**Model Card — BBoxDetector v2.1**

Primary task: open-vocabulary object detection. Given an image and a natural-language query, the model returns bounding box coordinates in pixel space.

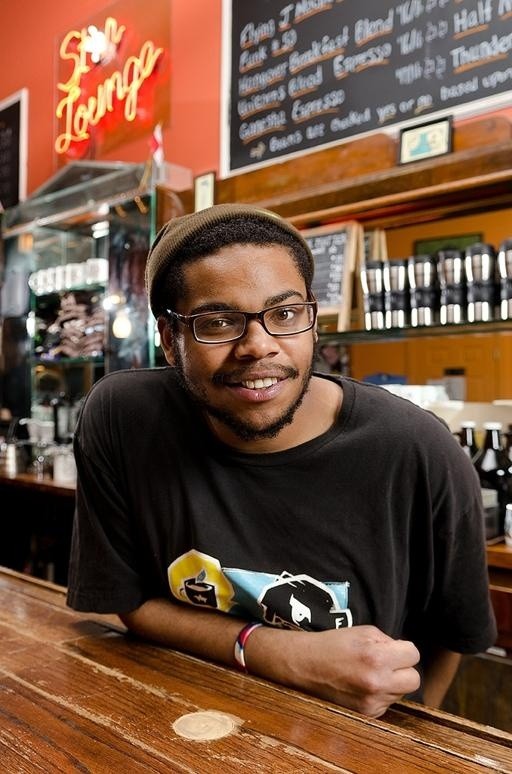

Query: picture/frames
[191,171,219,215]
[400,112,453,164]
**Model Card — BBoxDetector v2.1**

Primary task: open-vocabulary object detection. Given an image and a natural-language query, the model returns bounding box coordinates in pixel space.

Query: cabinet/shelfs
[278,173,510,565]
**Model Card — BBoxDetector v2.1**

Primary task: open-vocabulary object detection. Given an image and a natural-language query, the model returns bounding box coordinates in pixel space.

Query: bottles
[456,420,512,548]
[359,242,512,332]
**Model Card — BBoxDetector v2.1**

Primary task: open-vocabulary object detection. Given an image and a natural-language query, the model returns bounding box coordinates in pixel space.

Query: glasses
[162,294,320,344]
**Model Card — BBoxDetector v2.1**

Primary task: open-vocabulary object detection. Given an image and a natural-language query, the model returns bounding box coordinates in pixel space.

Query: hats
[146,203,316,317]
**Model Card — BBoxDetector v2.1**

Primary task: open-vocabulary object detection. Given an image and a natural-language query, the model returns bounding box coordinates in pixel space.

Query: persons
[68,205,500,721]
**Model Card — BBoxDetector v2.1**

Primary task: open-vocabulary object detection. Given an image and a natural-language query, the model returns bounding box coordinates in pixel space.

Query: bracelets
[234,620,264,676]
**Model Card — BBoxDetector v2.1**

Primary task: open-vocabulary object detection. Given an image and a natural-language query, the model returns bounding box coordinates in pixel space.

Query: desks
[0,568,512,773]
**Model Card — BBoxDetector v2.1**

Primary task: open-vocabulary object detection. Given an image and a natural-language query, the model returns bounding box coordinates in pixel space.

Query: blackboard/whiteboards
[218,0,512,182]
[298,219,379,317]
